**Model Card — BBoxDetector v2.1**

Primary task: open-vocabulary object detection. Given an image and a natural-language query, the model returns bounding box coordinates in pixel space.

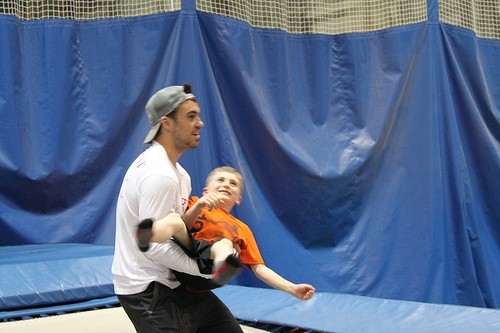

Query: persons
[136,166,315,301]
[111,83,245,333]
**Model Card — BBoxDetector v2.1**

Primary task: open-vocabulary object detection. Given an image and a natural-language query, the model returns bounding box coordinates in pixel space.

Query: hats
[144,86,196,144]
